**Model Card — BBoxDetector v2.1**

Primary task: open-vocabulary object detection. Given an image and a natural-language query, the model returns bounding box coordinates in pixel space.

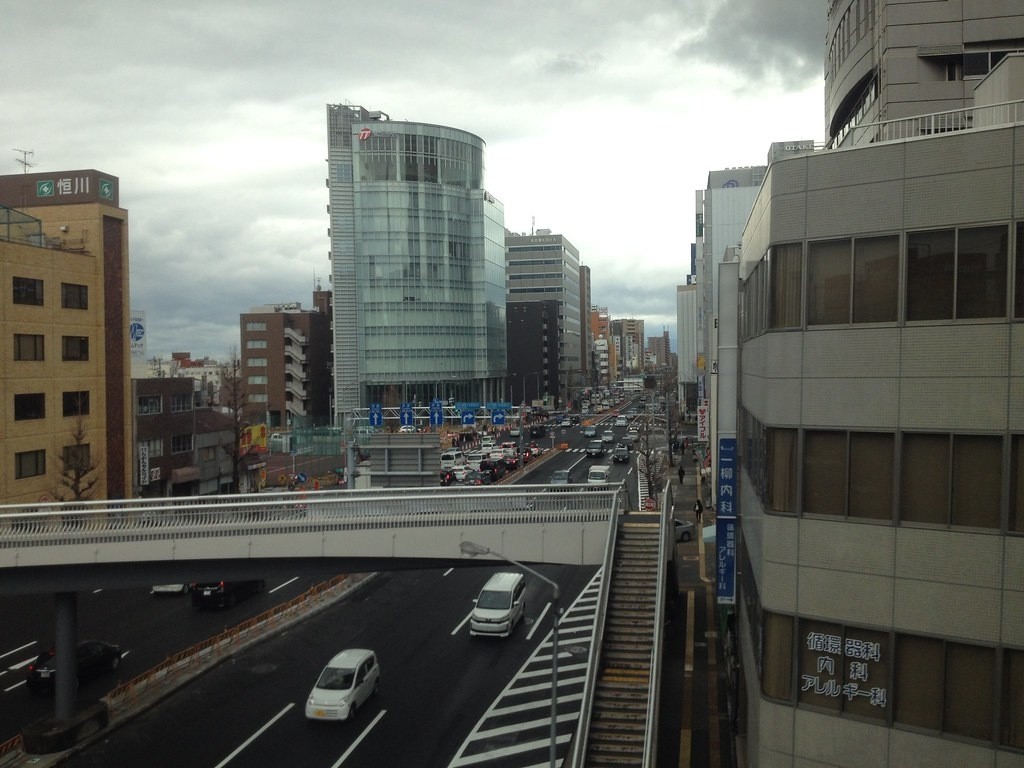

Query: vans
[305,648,380,723]
[469,573,528,638]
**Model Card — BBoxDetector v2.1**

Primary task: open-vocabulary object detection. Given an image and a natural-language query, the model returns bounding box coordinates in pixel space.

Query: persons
[678,466,685,484]
[674,437,698,461]
[693,499,704,523]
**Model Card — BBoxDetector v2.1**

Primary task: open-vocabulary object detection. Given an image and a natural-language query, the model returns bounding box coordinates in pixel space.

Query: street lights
[436,376,457,401]
[522,371,537,404]
[457,537,558,768]
[501,373,517,403]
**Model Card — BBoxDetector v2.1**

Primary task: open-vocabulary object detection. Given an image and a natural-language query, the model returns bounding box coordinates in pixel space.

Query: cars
[674,519,695,542]
[585,425,596,437]
[600,430,616,442]
[549,470,573,484]
[558,390,654,464]
[190,575,266,605]
[438,425,549,485]
[586,440,606,459]
[27,641,118,696]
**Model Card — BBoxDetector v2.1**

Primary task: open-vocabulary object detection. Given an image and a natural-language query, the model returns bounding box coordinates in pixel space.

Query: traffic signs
[401,403,412,426]
[430,402,444,427]
[369,404,384,428]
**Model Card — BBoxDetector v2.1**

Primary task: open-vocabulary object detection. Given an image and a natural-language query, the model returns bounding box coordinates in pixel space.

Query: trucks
[588,465,611,483]
